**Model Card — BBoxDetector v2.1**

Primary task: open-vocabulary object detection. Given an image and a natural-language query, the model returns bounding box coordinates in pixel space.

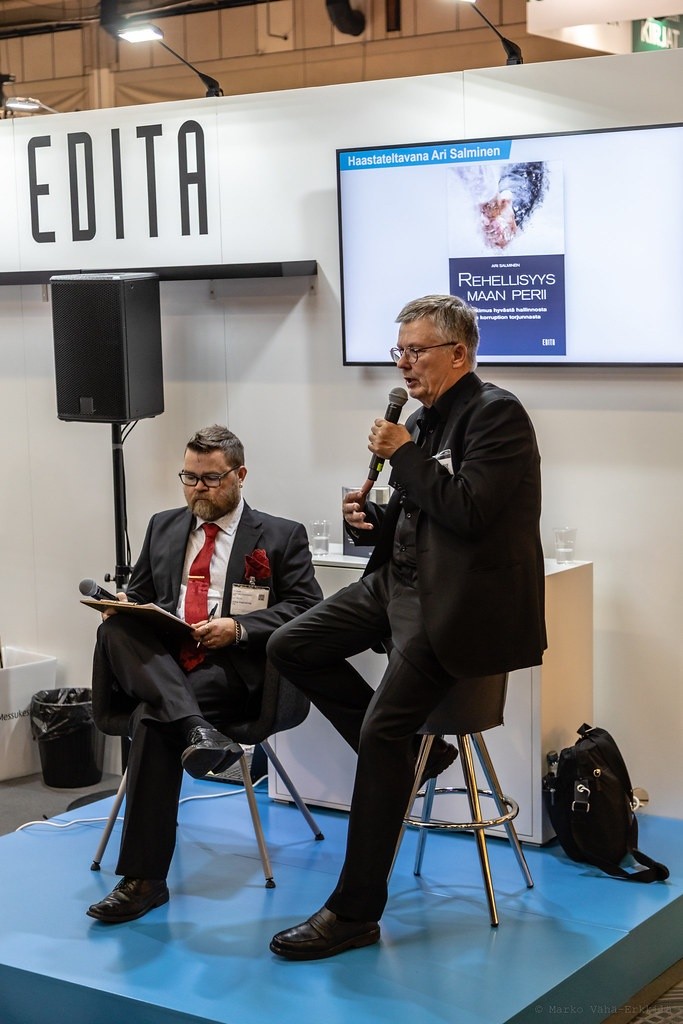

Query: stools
[386,672,534,927]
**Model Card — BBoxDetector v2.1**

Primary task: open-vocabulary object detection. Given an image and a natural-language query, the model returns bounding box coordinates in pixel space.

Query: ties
[180,522,223,672]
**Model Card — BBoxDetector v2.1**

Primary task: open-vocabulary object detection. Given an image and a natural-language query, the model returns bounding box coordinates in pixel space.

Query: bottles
[543,772,558,807]
[546,751,560,778]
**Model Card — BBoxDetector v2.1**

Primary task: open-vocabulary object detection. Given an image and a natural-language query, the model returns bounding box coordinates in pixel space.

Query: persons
[84,425,323,924]
[266,293,549,964]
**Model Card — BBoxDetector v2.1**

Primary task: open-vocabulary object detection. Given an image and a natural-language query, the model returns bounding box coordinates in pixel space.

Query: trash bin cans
[31,688,106,793]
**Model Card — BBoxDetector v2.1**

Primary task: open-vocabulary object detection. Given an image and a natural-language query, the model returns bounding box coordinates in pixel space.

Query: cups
[555,526,577,564]
[311,521,328,555]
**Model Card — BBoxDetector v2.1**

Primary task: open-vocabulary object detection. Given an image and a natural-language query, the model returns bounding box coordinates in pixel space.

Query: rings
[208,640,212,646]
[206,625,210,633]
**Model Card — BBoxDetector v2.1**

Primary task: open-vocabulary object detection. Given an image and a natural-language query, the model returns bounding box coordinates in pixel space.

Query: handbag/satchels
[541,722,670,883]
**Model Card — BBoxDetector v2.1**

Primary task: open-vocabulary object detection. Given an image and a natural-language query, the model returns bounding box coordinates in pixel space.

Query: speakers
[49,272,164,421]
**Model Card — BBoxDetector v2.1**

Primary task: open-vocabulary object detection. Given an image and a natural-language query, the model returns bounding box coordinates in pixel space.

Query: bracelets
[234,621,240,646]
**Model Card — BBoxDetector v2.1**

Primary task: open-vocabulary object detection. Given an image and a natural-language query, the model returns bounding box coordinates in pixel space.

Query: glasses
[389,343,457,363]
[178,464,242,487]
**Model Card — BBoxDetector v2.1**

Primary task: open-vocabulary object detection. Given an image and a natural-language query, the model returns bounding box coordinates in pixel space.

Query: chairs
[89,642,325,889]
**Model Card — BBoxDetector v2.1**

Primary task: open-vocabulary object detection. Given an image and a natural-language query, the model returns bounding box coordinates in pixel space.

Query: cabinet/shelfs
[266,542,594,847]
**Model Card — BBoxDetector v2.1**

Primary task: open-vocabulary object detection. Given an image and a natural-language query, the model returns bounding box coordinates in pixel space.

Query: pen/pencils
[196,603,219,649]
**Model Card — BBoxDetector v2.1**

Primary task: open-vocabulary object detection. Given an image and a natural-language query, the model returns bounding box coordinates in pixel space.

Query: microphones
[79,580,119,601]
[367,387,408,481]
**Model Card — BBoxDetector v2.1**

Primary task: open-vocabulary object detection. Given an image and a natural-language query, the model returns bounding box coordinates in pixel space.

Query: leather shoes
[86,875,169,921]
[415,734,458,792]
[269,906,381,960]
[180,726,244,779]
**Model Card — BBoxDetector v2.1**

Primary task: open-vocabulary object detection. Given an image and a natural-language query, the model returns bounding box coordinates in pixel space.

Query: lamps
[117,23,223,97]
[6,97,60,114]
